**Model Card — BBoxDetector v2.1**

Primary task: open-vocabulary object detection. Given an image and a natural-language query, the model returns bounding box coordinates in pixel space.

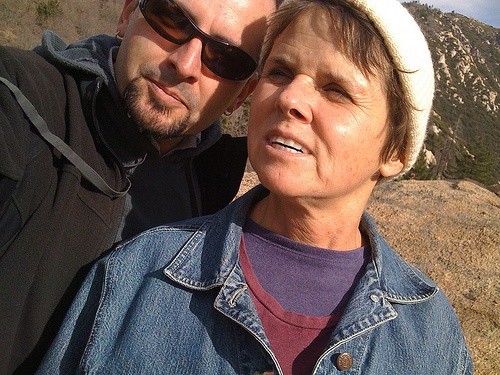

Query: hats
[259,0,436,177]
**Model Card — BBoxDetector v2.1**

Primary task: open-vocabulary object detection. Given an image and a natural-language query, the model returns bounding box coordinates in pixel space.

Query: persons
[0,0,282,375]
[35,0,473,375]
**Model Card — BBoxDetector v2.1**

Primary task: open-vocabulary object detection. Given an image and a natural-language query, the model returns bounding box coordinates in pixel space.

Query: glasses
[136,0,261,81]
